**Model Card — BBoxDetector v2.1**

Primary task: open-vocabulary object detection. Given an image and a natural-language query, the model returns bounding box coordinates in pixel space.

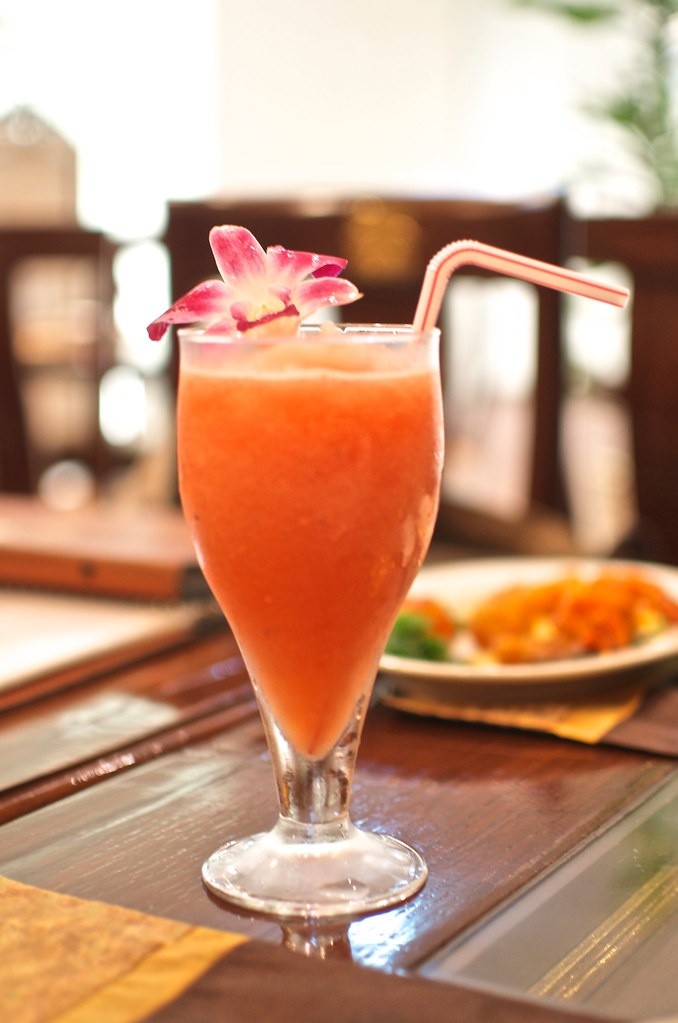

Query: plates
[352,557,678,696]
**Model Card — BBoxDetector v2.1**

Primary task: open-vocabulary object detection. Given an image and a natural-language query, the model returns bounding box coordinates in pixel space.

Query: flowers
[144,223,365,341]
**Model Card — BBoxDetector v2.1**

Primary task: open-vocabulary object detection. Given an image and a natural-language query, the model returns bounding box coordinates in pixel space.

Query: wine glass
[173,323,445,922]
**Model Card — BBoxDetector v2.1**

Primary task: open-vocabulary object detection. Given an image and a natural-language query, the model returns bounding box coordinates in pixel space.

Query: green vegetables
[386,614,453,661]
